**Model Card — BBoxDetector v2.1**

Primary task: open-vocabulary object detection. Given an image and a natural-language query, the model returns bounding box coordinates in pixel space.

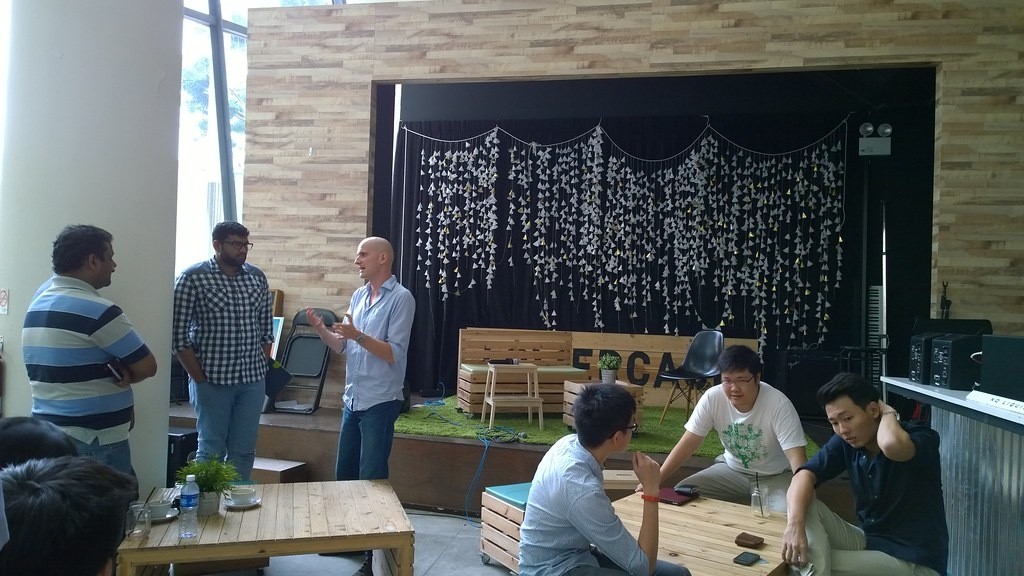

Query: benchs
[457,329,590,419]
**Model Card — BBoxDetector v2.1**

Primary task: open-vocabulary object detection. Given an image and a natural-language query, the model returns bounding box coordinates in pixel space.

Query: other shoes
[351,561,373,576]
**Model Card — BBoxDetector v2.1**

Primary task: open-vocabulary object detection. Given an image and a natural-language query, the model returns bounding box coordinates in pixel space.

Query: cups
[231,488,256,504]
[173,495,180,507]
[147,501,171,517]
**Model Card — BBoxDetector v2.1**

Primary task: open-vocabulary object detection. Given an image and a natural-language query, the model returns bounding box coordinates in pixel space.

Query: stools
[479,482,532,576]
[480,362,544,431]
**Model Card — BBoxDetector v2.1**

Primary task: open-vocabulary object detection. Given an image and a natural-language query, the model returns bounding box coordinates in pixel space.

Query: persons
[781,372,949,576]
[0,417,139,576]
[172,221,275,482]
[305,237,416,576]
[22,225,157,501]
[635,345,808,514]
[517,383,692,576]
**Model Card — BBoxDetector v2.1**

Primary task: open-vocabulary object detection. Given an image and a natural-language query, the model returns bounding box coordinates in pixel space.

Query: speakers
[167,426,198,487]
[785,349,863,421]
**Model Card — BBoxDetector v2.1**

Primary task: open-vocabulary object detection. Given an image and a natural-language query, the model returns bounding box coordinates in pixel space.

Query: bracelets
[641,495,659,502]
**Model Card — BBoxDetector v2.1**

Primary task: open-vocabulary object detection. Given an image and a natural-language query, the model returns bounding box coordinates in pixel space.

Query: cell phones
[733,552,760,566]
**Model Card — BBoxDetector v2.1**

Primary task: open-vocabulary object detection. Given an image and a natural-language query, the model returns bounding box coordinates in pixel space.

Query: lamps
[858,123,892,155]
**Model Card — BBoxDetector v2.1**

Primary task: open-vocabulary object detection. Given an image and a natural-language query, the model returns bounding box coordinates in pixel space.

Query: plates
[223,497,262,509]
[145,508,180,522]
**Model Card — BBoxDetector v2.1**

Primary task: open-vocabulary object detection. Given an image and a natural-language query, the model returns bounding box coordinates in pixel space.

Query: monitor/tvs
[981,334,1024,402]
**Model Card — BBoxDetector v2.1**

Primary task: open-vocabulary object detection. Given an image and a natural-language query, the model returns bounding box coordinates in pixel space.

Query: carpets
[394,394,820,463]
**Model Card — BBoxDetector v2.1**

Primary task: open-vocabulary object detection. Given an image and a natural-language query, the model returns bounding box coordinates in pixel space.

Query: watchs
[881,410,901,423]
[356,333,365,346]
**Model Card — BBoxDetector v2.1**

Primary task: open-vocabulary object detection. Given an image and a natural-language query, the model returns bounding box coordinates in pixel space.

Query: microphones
[487,359,519,364]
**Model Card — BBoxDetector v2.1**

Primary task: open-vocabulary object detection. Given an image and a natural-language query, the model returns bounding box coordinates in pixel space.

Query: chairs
[269,308,338,415]
[658,331,724,426]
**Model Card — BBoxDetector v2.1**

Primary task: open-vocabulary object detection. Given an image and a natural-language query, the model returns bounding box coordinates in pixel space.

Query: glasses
[722,373,756,386]
[218,239,254,249]
[609,421,639,439]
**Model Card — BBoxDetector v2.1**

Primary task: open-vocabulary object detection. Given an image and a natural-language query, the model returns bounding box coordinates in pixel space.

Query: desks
[563,380,644,439]
[611,490,800,576]
[115,479,415,576]
[879,375,1024,576]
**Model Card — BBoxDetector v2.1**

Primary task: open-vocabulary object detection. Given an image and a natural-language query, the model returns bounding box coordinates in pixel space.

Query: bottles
[126,500,151,539]
[178,474,199,537]
[751,486,771,518]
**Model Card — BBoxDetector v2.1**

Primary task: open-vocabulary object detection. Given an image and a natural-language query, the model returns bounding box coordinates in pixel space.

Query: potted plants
[173,450,243,516]
[597,352,621,384]
